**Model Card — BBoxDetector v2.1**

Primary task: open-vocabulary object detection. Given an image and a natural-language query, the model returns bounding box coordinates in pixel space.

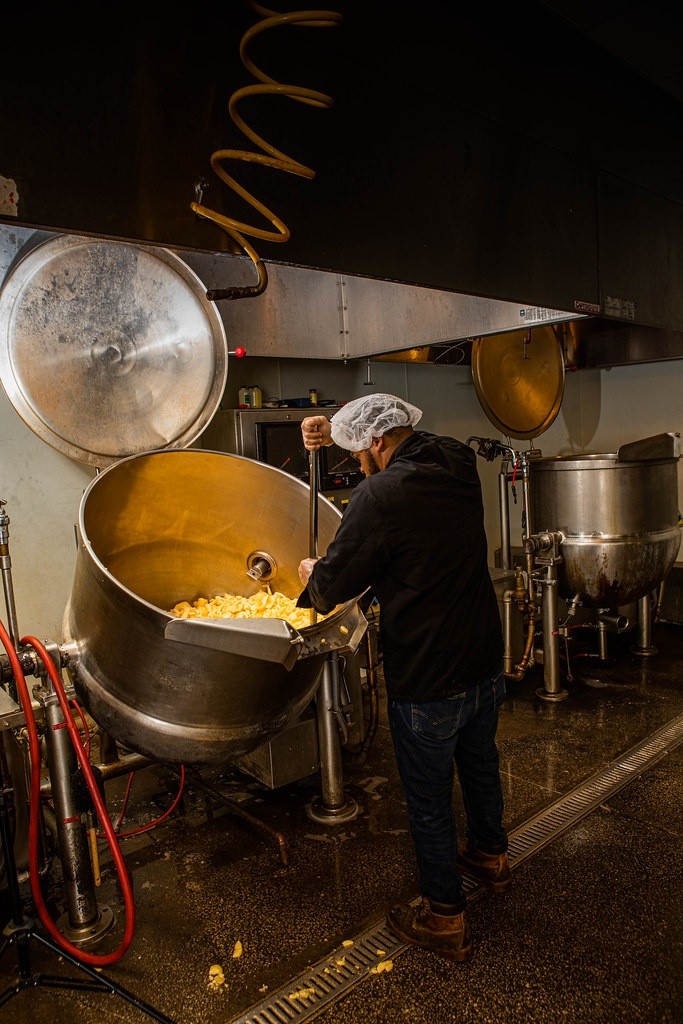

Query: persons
[295,393,514,963]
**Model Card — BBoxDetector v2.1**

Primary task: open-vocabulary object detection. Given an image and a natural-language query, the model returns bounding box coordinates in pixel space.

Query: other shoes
[456,839,514,892]
[387,896,473,962]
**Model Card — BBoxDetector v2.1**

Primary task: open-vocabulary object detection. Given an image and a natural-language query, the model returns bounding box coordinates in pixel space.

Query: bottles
[239,385,262,409]
